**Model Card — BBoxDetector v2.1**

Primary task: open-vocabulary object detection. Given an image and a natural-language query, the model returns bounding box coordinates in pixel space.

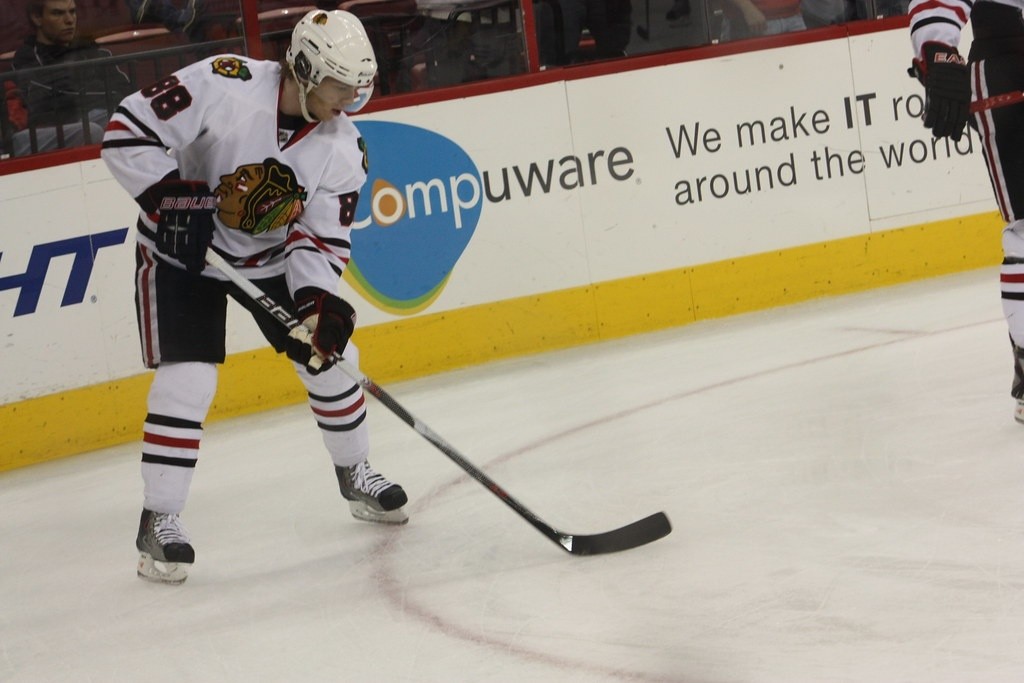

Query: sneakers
[1012,346,1024,425]
[334,458,409,525]
[136,508,195,585]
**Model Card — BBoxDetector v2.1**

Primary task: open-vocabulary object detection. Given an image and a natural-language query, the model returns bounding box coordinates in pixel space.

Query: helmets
[286,9,378,96]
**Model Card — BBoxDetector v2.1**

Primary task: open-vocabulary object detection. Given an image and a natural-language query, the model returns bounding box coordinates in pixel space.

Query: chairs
[0,0,427,131]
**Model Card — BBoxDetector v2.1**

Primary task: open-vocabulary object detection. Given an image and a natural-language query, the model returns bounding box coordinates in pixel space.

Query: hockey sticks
[970,90,1024,116]
[201,246,676,558]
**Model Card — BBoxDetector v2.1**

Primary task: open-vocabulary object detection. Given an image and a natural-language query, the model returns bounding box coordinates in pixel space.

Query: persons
[98,10,408,585]
[0,0,431,198]
[721,0,805,45]
[908,0,1024,429]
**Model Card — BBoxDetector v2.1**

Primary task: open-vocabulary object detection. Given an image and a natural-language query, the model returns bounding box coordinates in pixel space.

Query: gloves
[148,179,216,276]
[907,41,972,142]
[287,292,357,376]
